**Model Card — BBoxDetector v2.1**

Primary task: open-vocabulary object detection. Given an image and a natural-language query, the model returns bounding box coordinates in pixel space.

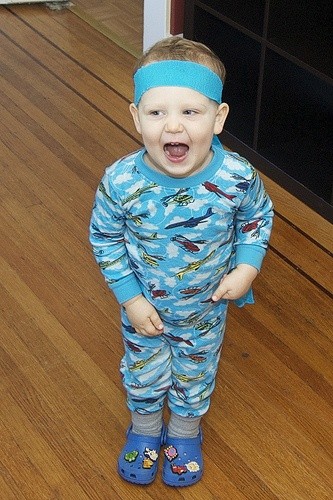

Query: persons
[88,36,274,484]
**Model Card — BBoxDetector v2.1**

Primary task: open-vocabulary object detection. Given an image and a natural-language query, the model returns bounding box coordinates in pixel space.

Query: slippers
[117,418,167,483]
[162,423,205,486]
